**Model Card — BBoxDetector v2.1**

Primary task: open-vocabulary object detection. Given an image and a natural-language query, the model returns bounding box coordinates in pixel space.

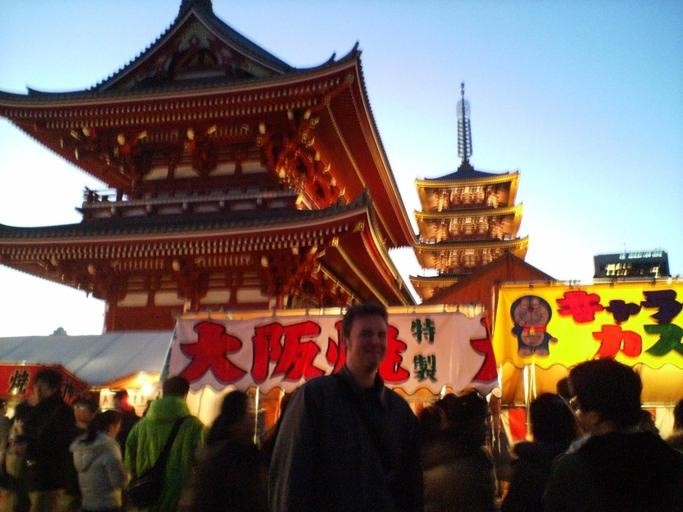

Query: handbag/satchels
[127,466,163,506]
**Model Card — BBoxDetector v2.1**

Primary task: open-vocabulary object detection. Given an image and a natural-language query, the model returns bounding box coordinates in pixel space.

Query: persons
[0,302,683,511]
